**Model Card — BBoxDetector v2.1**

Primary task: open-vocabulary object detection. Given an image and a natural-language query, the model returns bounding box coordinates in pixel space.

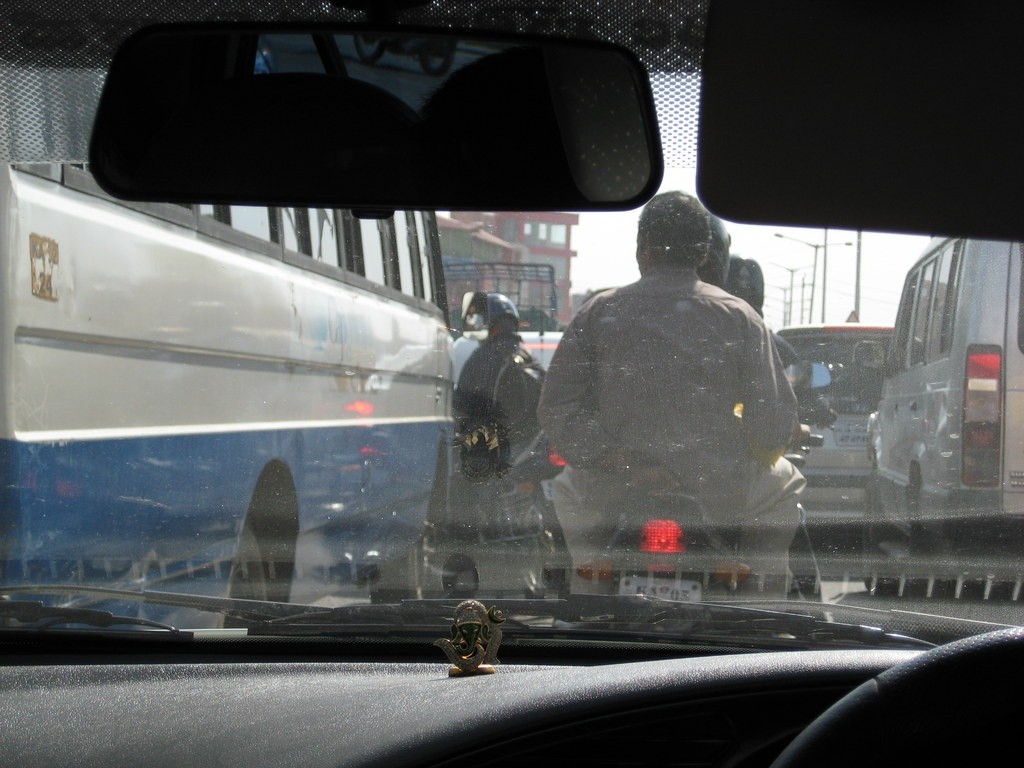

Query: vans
[866,228,1024,517]
[774,323,905,516]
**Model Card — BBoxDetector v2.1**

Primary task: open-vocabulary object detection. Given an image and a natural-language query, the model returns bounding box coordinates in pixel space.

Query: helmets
[636,190,712,270]
[487,292,520,332]
[706,214,730,287]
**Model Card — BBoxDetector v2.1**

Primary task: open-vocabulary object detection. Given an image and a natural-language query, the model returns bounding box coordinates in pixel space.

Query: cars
[449,324,573,386]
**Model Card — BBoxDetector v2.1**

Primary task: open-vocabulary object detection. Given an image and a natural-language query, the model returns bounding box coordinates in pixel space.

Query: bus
[2,42,473,636]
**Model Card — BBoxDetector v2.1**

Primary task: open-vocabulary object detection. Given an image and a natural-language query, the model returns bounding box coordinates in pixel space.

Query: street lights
[773,231,853,325]
[770,261,810,326]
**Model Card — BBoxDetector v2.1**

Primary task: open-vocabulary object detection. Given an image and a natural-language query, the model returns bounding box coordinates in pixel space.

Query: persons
[466,306,483,325]
[449,293,534,586]
[536,192,821,625]
